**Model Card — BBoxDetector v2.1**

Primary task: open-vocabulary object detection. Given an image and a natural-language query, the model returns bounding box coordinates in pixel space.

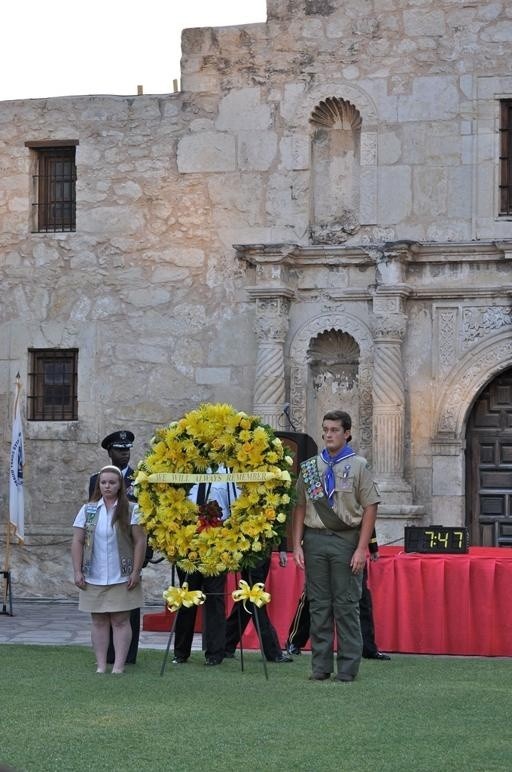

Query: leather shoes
[310,670,355,681]
[170,653,220,670]
[270,639,302,663]
[366,652,391,660]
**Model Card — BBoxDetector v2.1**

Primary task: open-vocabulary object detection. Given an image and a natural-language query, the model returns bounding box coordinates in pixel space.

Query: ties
[196,483,206,506]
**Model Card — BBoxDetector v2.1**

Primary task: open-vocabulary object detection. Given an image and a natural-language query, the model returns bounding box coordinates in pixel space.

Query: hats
[101,431,135,449]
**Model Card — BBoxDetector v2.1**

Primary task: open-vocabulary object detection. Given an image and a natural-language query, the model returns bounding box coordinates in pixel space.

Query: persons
[69,464,147,677]
[87,426,155,666]
[282,526,390,660]
[168,458,241,668]
[219,547,290,663]
[291,408,380,684]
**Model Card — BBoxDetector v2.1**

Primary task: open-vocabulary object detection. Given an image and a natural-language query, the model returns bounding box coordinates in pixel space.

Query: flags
[9,382,26,545]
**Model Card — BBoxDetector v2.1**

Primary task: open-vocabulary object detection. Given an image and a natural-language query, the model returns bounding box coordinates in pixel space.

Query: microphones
[281,402,291,415]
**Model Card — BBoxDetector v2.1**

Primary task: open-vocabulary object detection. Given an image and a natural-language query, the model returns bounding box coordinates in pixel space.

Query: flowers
[134,403,293,577]
